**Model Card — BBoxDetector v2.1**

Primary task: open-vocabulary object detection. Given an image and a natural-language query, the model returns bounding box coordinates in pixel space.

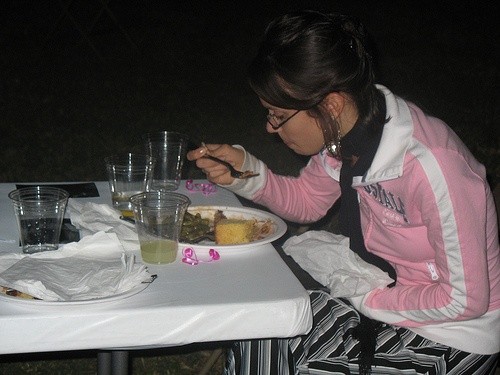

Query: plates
[143,205,288,249]
[0,253,155,304]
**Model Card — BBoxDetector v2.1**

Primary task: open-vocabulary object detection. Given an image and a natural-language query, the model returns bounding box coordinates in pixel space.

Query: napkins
[0,197,150,303]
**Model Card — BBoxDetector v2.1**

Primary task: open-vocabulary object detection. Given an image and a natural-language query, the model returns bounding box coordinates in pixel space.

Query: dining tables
[0,178,313,375]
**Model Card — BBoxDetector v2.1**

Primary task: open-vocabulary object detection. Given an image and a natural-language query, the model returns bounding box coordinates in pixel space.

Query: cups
[129,191,190,264]
[142,130,189,191]
[104,153,153,213]
[8,186,69,254]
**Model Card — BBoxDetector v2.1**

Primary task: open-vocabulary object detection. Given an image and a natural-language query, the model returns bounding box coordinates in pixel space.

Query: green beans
[146,210,217,244]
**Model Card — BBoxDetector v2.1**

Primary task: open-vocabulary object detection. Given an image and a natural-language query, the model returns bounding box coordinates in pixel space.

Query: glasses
[259,105,301,130]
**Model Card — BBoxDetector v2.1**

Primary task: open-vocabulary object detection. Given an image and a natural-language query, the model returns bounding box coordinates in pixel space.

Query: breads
[214,218,255,244]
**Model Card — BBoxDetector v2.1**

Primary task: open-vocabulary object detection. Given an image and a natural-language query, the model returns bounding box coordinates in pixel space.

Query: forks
[202,154,260,179]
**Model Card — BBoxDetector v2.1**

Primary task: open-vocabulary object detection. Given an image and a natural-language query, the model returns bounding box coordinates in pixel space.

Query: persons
[187,11,500,375]
